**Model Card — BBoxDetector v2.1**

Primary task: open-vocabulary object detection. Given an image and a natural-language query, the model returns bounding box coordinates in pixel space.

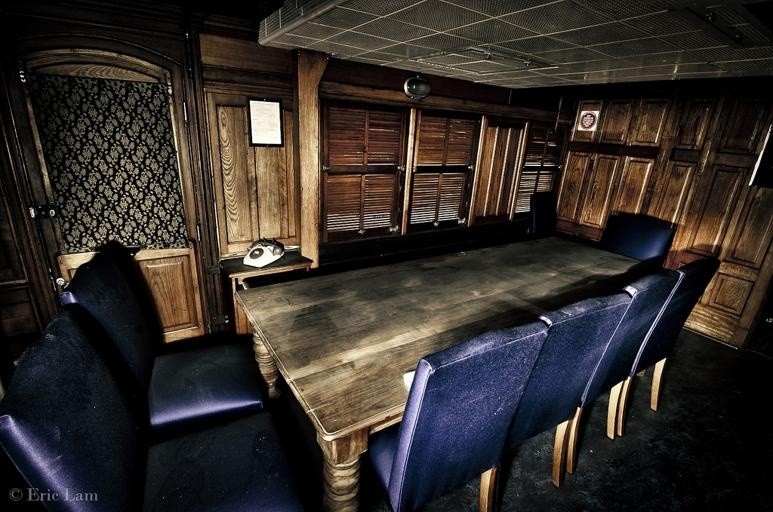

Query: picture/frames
[246,95,285,148]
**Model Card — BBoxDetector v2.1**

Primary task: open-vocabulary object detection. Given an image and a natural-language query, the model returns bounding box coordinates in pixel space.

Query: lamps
[402,70,432,101]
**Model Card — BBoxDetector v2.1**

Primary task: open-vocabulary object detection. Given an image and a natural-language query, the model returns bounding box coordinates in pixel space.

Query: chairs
[0,194,721,508]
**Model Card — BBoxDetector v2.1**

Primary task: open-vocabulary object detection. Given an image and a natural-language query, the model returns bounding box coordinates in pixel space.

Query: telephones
[242,239,284,268]
